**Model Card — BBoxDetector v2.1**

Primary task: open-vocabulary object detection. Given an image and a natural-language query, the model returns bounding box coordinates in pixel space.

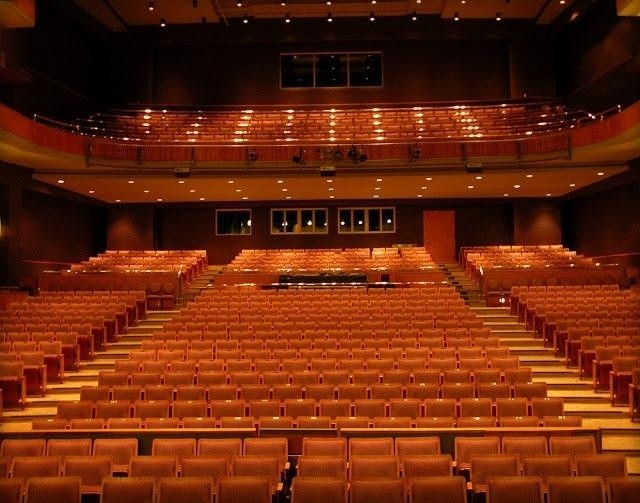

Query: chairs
[61,251,208,286]
[0,285,639,501]
[458,245,623,306]
[219,247,440,274]
[111,104,581,144]
